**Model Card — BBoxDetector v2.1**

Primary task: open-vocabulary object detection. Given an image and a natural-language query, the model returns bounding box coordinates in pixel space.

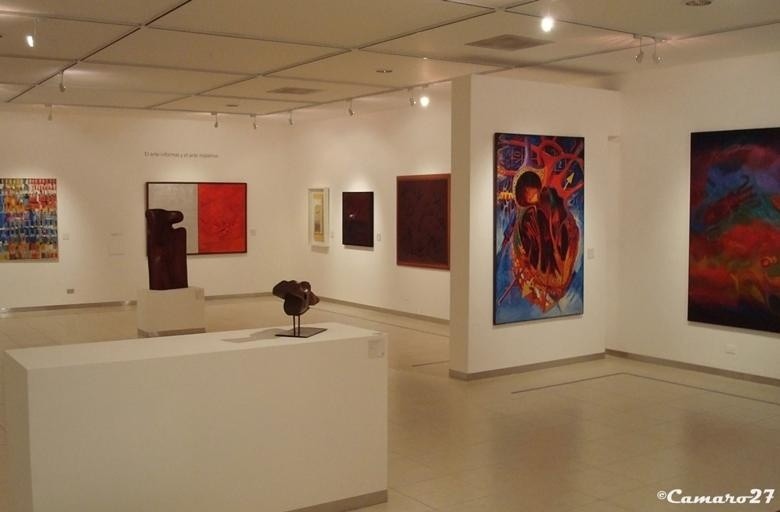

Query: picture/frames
[307,187,331,247]
[397,174,454,271]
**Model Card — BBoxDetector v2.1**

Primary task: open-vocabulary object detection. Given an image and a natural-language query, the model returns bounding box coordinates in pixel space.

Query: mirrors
[146,180,248,256]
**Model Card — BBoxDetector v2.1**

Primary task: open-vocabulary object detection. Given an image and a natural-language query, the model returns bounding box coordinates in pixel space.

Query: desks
[1,323,388,510]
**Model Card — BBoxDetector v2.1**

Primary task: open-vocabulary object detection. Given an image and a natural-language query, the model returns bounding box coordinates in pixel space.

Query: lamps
[288,109,295,128]
[408,88,417,106]
[48,105,54,122]
[60,71,66,92]
[349,99,353,117]
[214,113,219,129]
[30,17,39,49]
[253,116,258,130]
[653,40,664,65]
[635,35,645,64]
